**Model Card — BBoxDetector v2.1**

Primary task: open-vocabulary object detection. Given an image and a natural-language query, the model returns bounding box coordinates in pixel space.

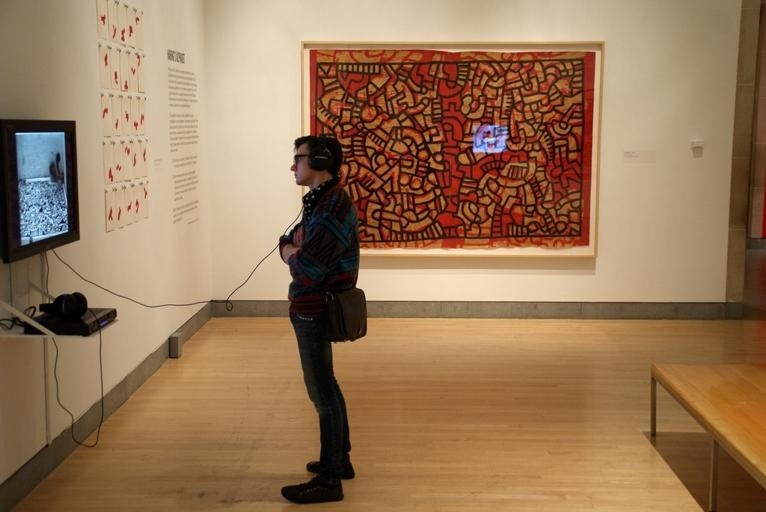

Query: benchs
[649,364,766,512]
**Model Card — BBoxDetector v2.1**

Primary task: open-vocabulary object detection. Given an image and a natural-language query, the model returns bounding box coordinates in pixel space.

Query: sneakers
[281,461,355,504]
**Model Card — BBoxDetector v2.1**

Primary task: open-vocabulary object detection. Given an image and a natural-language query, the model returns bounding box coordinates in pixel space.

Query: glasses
[294,154,308,162]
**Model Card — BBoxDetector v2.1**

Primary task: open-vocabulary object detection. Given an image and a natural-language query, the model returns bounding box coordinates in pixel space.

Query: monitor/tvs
[0,120,80,264]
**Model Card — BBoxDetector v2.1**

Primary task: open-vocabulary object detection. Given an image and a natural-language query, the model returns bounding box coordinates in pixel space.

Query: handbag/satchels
[323,288,367,343]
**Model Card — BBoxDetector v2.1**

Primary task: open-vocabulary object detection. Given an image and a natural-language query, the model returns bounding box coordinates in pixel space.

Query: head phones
[309,133,333,171]
[40,292,87,318]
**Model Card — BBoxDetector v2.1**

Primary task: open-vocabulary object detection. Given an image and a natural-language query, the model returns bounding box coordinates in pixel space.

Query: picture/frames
[299,41,605,260]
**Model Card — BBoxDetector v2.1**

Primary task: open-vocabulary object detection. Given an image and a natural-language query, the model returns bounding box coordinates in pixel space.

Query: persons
[49,151,64,184]
[279,135,369,505]
